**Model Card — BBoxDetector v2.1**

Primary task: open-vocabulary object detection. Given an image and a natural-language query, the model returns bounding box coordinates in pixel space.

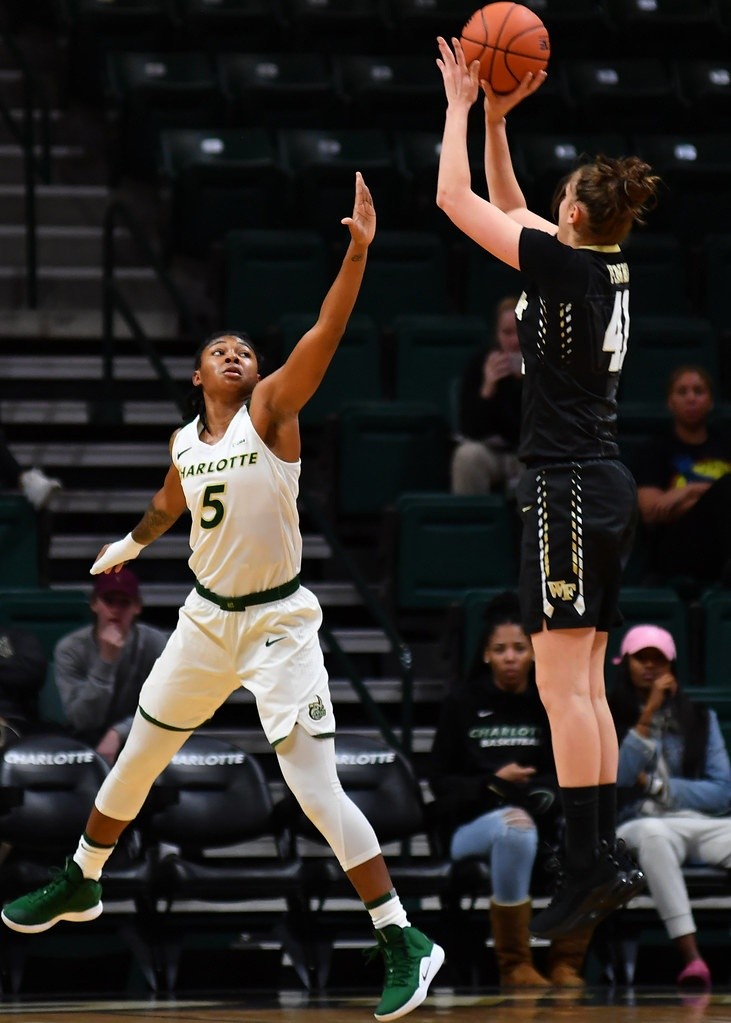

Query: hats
[612,625,677,665]
[94,569,139,604]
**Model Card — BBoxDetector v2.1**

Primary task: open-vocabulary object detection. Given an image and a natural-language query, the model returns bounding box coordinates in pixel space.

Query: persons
[451,298,520,498]
[639,365,730,591]
[0,170,446,1022]
[41,566,196,884]
[434,34,659,938]
[433,619,588,986]
[0,628,48,720]
[611,623,731,984]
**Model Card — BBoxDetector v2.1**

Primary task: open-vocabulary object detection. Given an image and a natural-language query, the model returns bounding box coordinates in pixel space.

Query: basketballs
[457,1,553,94]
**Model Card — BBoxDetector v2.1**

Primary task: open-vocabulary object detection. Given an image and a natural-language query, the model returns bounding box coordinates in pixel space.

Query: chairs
[0,3,728,989]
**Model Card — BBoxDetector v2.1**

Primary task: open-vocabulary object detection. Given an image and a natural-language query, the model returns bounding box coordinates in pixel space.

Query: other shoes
[679,959,710,986]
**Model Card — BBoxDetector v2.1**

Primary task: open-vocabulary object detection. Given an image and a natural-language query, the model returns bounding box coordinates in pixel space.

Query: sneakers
[367,924,446,1021]
[1,855,104,934]
[527,839,625,935]
[556,845,645,938]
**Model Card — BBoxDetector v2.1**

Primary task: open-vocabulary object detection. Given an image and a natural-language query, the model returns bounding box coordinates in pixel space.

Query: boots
[551,921,592,988]
[491,892,550,988]
